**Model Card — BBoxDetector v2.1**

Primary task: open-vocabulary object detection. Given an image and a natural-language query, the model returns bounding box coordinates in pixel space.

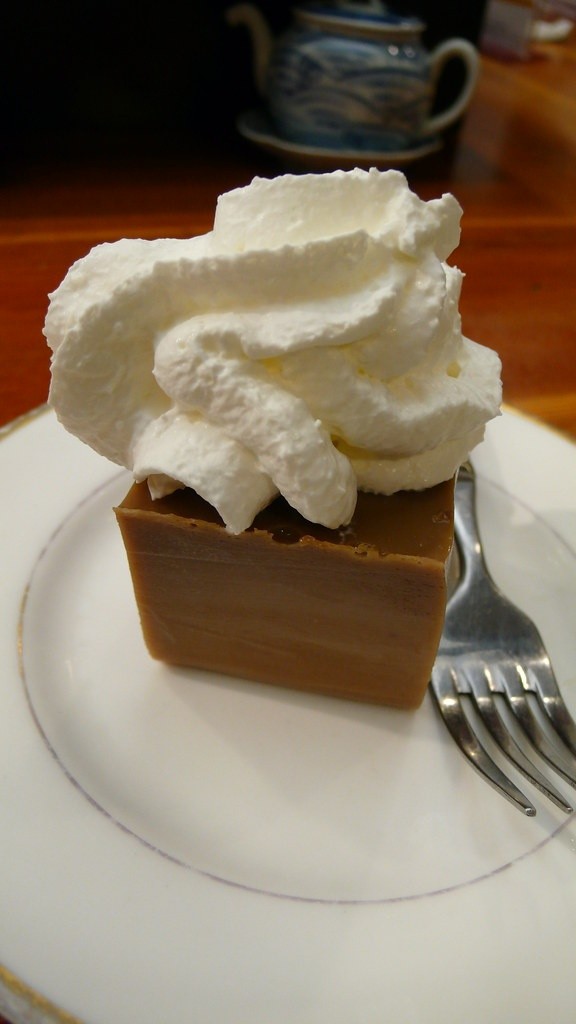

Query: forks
[426,457,575,820]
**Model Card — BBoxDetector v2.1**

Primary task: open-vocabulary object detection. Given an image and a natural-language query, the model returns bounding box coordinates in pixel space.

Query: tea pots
[226,0,483,154]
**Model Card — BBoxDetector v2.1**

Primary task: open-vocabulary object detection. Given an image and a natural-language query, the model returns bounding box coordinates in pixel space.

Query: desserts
[42,165,503,710]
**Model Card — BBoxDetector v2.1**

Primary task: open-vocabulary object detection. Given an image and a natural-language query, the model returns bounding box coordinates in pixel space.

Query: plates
[236,117,445,171]
[1,395,576,1024]
